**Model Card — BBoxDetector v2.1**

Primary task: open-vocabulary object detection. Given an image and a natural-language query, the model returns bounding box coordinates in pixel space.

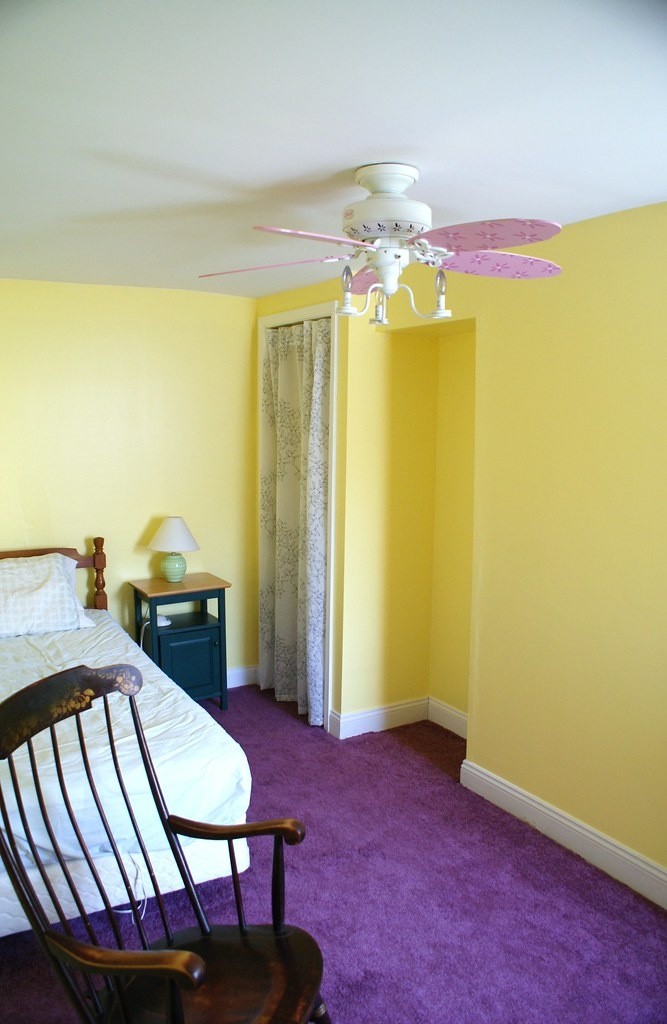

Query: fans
[198,161,563,295]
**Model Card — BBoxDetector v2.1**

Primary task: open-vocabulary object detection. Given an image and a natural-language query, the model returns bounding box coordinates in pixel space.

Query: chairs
[0,664,331,1024]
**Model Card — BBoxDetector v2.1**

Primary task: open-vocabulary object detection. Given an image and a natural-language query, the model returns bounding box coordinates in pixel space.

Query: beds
[0,536,252,938]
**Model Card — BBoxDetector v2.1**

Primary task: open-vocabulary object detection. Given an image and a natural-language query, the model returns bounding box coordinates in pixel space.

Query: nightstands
[128,571,232,711]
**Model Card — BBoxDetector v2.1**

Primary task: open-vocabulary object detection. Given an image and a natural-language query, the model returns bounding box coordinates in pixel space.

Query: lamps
[335,247,451,324]
[146,515,200,583]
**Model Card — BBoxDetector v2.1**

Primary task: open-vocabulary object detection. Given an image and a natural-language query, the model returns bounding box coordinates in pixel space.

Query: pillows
[0,552,96,639]
